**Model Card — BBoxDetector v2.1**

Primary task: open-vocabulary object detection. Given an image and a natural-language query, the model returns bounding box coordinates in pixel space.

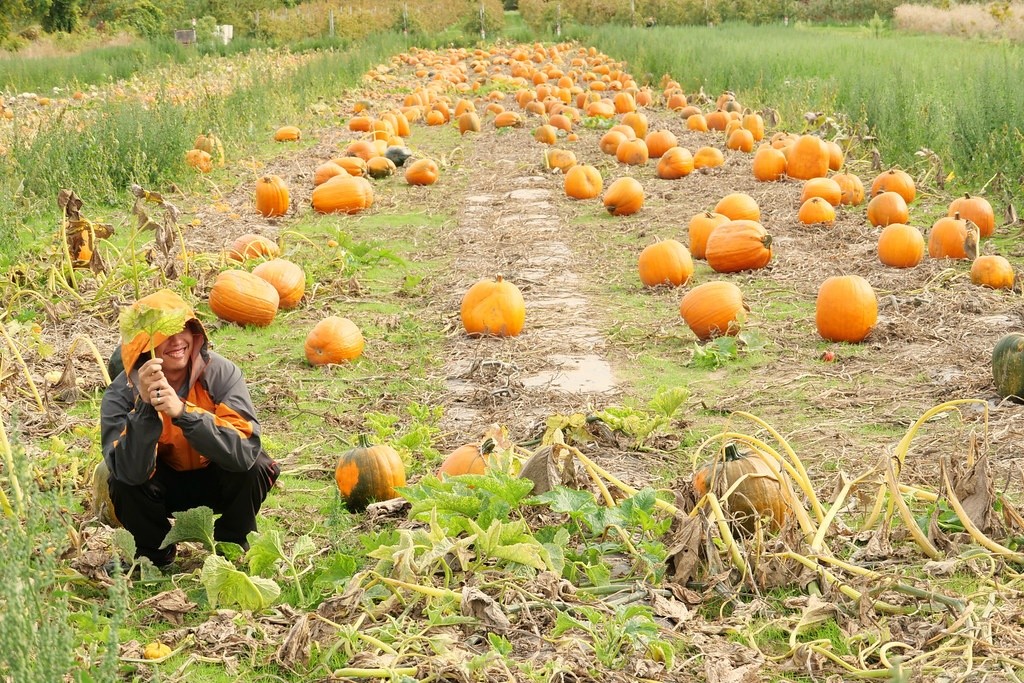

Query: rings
[156,389,161,404]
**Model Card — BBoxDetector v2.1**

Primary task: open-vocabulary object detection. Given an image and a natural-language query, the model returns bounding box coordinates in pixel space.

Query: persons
[101,289,282,577]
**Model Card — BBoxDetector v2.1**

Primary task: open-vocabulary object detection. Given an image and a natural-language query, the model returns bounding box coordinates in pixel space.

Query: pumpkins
[185,39,1024,513]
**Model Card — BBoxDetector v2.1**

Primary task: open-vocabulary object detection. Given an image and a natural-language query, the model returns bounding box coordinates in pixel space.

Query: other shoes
[134,542,177,567]
